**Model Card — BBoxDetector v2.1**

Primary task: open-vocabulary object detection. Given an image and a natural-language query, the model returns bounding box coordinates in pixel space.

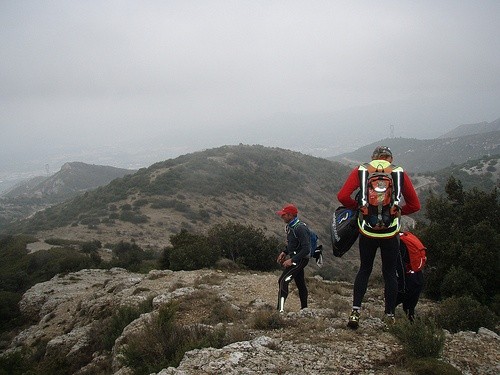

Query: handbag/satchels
[331,206,363,257]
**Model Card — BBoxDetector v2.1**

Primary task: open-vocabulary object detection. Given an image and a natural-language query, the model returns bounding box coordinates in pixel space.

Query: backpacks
[360,163,400,230]
[292,221,323,269]
[399,231,427,273]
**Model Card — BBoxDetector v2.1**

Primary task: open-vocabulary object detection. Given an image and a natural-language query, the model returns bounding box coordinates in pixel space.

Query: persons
[398,230,426,323]
[337,145,422,330]
[275,204,311,313]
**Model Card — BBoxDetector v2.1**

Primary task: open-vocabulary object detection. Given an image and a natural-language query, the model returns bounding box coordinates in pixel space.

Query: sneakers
[346,308,360,329]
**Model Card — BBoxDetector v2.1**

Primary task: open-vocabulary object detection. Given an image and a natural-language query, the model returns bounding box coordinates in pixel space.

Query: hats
[372,146,392,156]
[276,205,298,215]
